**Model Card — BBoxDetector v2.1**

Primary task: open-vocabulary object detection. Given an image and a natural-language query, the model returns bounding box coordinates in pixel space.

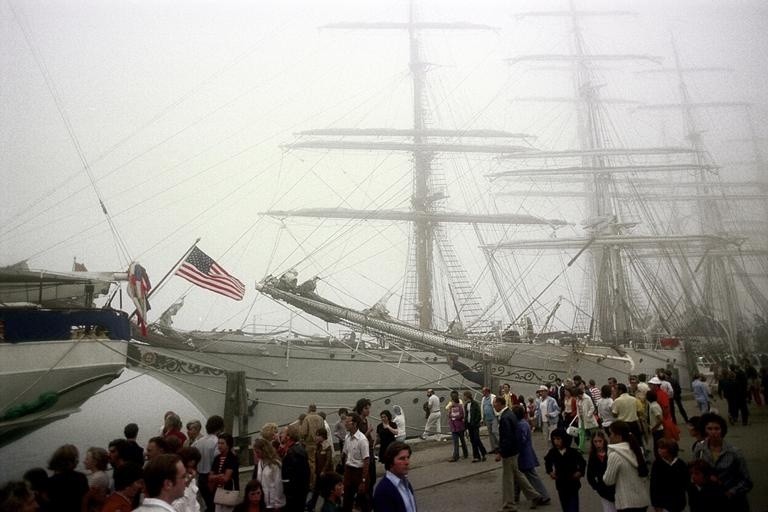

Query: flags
[175,245,246,302]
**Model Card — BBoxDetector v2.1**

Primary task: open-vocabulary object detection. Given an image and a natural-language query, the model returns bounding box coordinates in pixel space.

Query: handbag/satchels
[567,415,580,437]
[213,479,241,506]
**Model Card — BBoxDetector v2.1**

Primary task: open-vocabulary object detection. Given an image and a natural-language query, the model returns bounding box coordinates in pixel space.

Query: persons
[299,276,321,292]
[277,270,299,292]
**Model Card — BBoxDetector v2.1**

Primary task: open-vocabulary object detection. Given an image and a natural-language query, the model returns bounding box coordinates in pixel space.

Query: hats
[537,385,548,392]
[647,376,662,385]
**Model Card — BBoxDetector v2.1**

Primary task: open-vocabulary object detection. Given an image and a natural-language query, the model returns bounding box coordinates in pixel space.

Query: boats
[0,261,129,449]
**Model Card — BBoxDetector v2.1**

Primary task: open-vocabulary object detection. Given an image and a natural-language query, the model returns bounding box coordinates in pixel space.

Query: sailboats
[127,0,768,465]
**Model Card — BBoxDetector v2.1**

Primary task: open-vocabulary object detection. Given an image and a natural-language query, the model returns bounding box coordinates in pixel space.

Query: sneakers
[494,452,501,462]
[497,494,551,511]
[446,453,488,462]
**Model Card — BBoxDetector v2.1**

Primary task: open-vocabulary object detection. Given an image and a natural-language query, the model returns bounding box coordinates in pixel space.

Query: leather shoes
[488,450,495,455]
